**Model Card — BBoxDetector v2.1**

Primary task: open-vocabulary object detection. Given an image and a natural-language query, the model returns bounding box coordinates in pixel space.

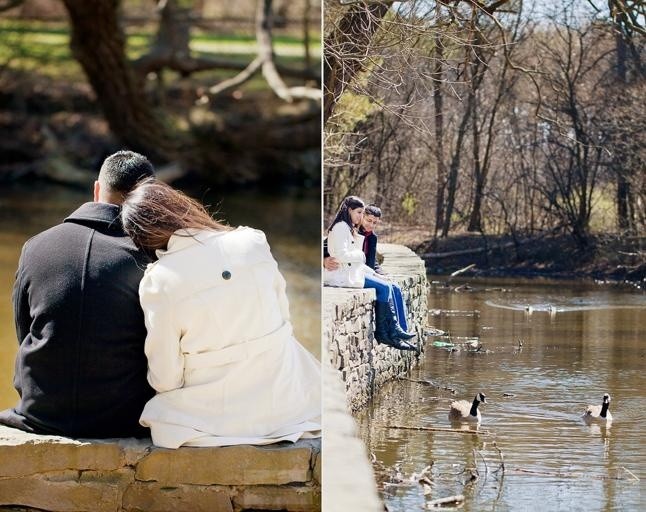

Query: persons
[324,195,419,350]
[1,150,322,449]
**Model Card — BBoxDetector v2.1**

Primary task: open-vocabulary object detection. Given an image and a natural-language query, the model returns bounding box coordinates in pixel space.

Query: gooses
[584,393,612,419]
[448,392,488,423]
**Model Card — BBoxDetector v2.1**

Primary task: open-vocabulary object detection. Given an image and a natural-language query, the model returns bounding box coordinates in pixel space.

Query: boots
[373,300,418,351]
